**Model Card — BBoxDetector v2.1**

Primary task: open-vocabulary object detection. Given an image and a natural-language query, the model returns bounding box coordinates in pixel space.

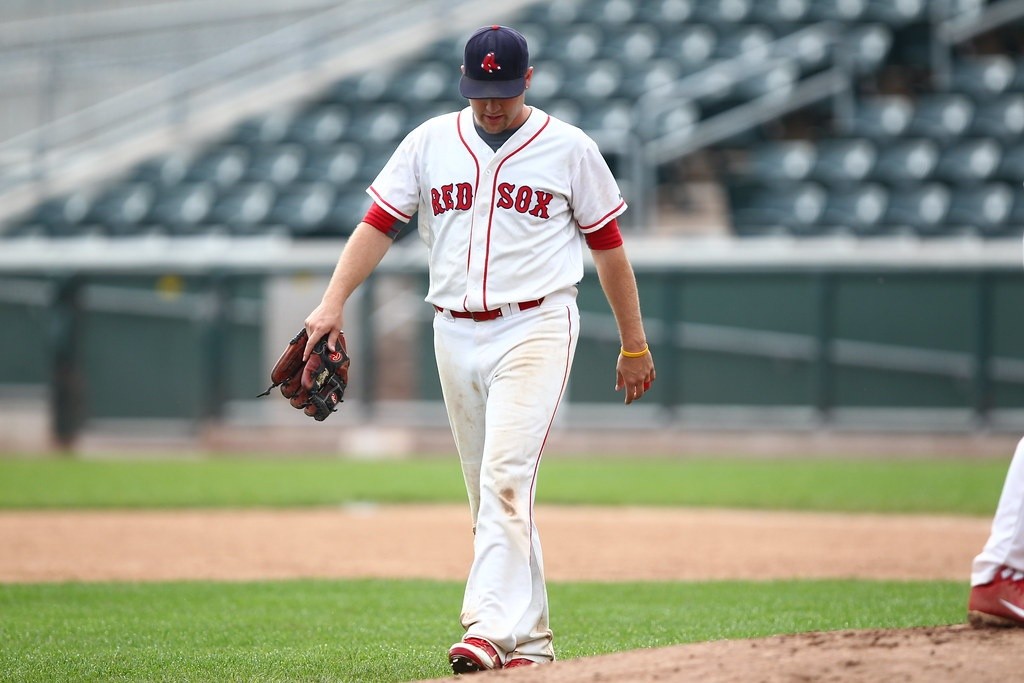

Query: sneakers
[502,656,538,668]
[968,564,1024,625]
[448,637,500,675]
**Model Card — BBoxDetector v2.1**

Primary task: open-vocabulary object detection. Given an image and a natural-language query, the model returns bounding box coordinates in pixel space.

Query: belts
[432,295,547,322]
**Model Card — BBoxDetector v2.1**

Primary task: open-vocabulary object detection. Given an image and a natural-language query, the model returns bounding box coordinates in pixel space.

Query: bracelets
[620,342,650,357]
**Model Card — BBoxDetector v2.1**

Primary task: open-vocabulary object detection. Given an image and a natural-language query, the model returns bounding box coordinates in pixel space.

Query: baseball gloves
[270,326,351,422]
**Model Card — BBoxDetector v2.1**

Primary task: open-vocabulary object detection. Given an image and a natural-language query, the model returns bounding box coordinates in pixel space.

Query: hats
[459,24,528,99]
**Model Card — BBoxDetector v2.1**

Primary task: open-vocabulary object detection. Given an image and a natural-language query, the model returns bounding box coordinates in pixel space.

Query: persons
[302,24,658,673]
[967,437,1023,629]
